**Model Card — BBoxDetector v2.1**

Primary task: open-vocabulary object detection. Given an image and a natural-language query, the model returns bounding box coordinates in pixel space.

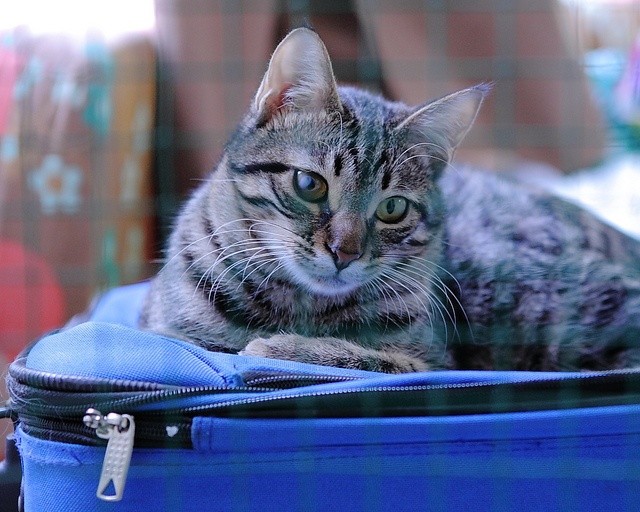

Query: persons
[154,0,607,189]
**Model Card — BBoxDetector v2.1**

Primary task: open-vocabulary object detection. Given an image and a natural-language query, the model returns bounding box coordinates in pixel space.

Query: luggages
[0,281,639,512]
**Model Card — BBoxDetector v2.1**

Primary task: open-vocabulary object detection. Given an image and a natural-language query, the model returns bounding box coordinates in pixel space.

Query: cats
[135,27,639,375]
